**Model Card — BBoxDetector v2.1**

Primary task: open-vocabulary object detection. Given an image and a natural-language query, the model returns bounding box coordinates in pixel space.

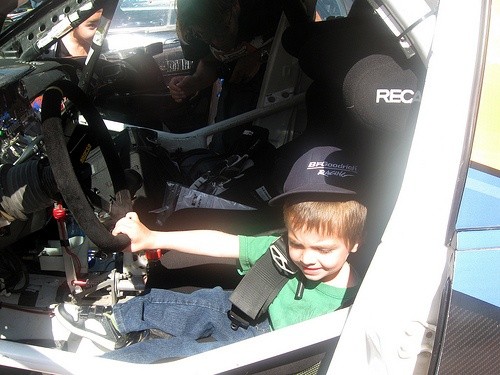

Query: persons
[55,146,370,364]
[162,0,305,132]
[58,8,108,57]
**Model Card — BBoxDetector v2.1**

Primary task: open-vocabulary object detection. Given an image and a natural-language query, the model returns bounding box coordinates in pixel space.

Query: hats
[269,146,374,205]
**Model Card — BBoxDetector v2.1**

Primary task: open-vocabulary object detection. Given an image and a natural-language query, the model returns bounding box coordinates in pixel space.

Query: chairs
[145,54,417,364]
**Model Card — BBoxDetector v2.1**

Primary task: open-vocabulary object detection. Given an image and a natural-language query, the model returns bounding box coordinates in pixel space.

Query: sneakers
[55,302,131,350]
